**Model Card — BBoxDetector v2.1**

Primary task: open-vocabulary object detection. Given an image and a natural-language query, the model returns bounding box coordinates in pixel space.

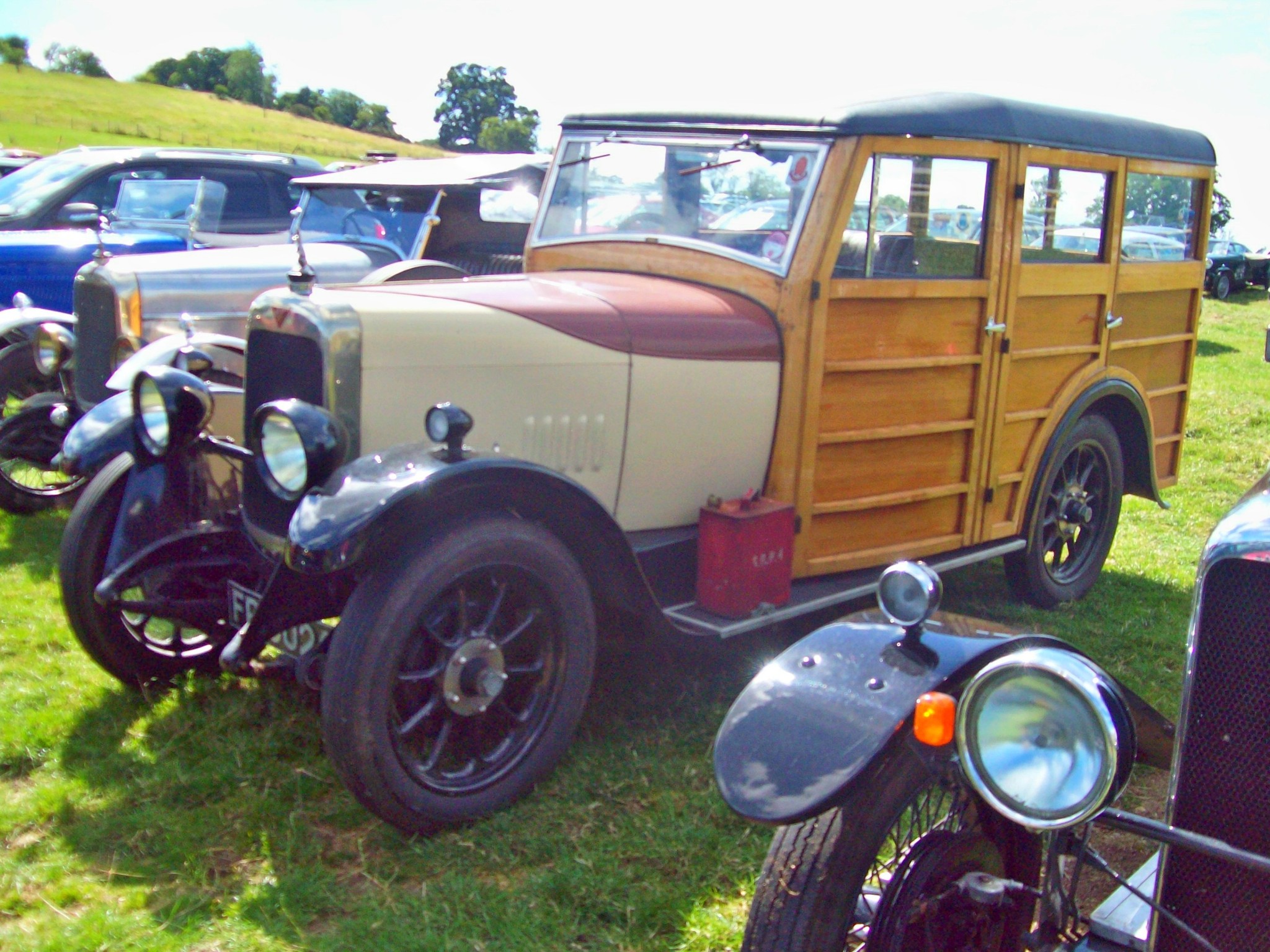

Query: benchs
[422,252,527,277]
[832,229,1102,285]
[450,242,524,258]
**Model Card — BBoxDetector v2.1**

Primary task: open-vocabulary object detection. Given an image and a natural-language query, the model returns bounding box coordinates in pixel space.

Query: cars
[6,111,709,558]
[548,175,1259,303]
[705,460,1270,950]
[48,72,1218,841]
